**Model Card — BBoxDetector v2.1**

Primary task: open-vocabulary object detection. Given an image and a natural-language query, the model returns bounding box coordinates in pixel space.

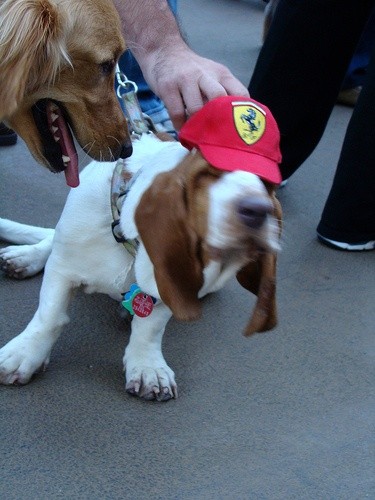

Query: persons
[111,0,249,139]
[248,0,375,253]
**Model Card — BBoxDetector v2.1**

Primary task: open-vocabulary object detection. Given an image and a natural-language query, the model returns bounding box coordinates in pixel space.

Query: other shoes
[147,106,178,139]
[0,121,18,145]
[316,233,375,250]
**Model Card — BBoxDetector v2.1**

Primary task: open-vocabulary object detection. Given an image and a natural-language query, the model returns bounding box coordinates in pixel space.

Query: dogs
[0,130,282,402]
[0,0,133,189]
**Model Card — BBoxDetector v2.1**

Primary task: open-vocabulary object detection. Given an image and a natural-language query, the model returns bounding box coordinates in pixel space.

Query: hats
[178,96,282,184]
